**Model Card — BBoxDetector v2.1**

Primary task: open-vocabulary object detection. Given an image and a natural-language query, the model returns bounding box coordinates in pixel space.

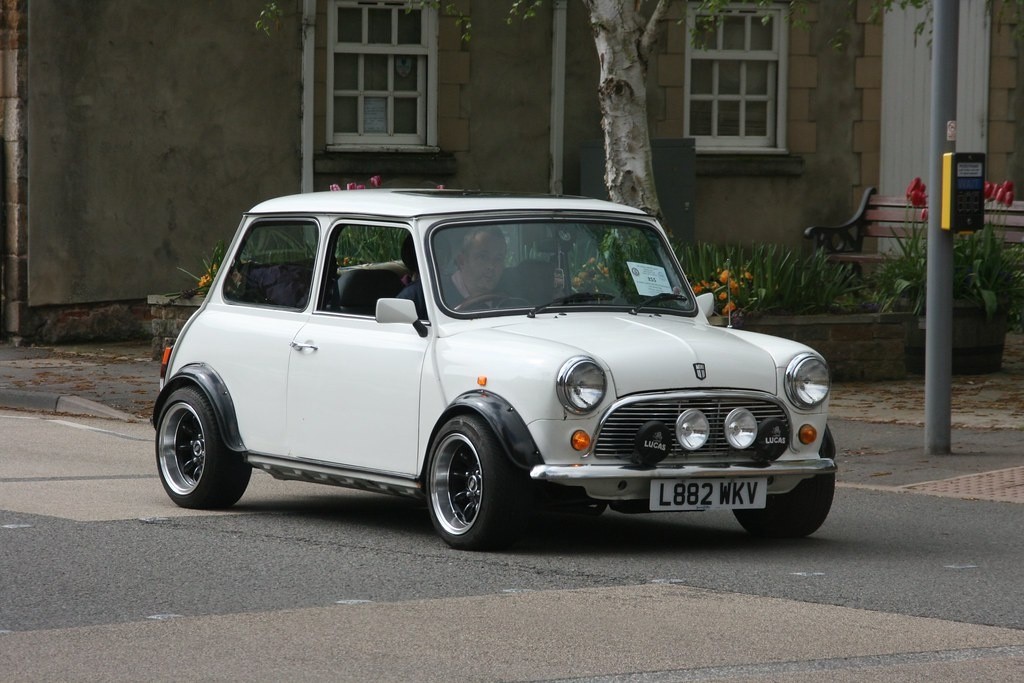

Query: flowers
[198,257,222,292]
[887,177,1020,311]
[571,257,628,296]
[694,262,763,325]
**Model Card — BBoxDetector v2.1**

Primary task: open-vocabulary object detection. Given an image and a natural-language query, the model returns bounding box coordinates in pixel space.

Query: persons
[399,228,453,289]
[394,225,533,320]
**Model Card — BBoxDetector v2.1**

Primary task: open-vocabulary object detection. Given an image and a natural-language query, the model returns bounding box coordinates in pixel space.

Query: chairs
[329,268,405,319]
[804,187,1024,293]
[494,255,554,308]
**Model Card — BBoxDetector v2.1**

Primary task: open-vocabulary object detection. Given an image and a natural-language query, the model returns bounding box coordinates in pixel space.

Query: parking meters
[941,151,986,236]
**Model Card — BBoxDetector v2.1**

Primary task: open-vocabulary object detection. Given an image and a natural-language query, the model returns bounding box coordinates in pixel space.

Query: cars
[149,190,839,550]
